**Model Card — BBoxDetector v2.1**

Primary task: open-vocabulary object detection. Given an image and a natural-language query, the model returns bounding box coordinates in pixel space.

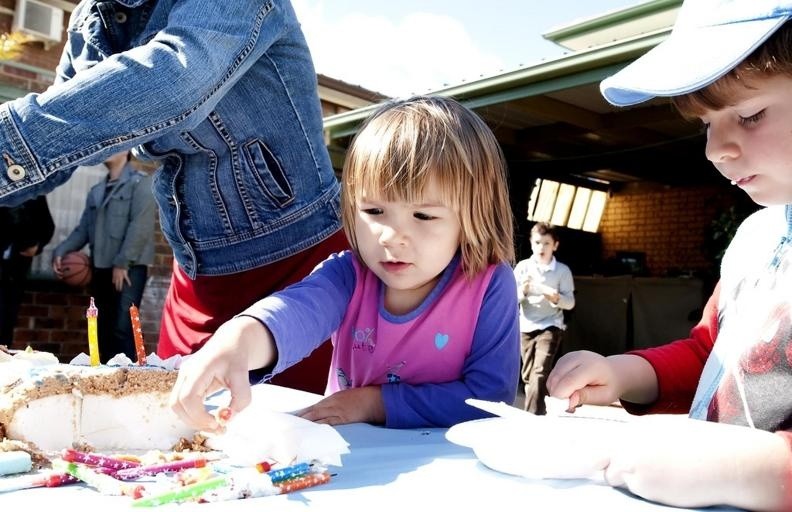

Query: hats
[600,0,792,107]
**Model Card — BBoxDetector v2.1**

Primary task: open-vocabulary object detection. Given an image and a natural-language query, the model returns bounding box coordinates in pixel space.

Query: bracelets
[775,426,792,511]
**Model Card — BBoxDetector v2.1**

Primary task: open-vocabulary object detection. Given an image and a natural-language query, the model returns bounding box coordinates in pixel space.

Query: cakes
[0,366,188,451]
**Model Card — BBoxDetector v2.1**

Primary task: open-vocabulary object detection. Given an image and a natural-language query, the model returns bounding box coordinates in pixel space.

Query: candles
[130,302,147,366]
[83,295,102,366]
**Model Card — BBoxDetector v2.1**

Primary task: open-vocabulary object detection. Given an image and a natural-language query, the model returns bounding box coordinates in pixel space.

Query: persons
[513,220,577,416]
[546,0,792,511]
[53,148,155,361]
[1,190,55,349]
[168,95,522,438]
[0,0,349,396]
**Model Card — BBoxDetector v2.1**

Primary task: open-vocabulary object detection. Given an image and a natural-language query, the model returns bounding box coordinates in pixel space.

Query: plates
[442,413,630,482]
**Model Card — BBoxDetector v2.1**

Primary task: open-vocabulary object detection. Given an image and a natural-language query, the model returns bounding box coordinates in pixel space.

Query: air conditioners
[11,0,64,51]
